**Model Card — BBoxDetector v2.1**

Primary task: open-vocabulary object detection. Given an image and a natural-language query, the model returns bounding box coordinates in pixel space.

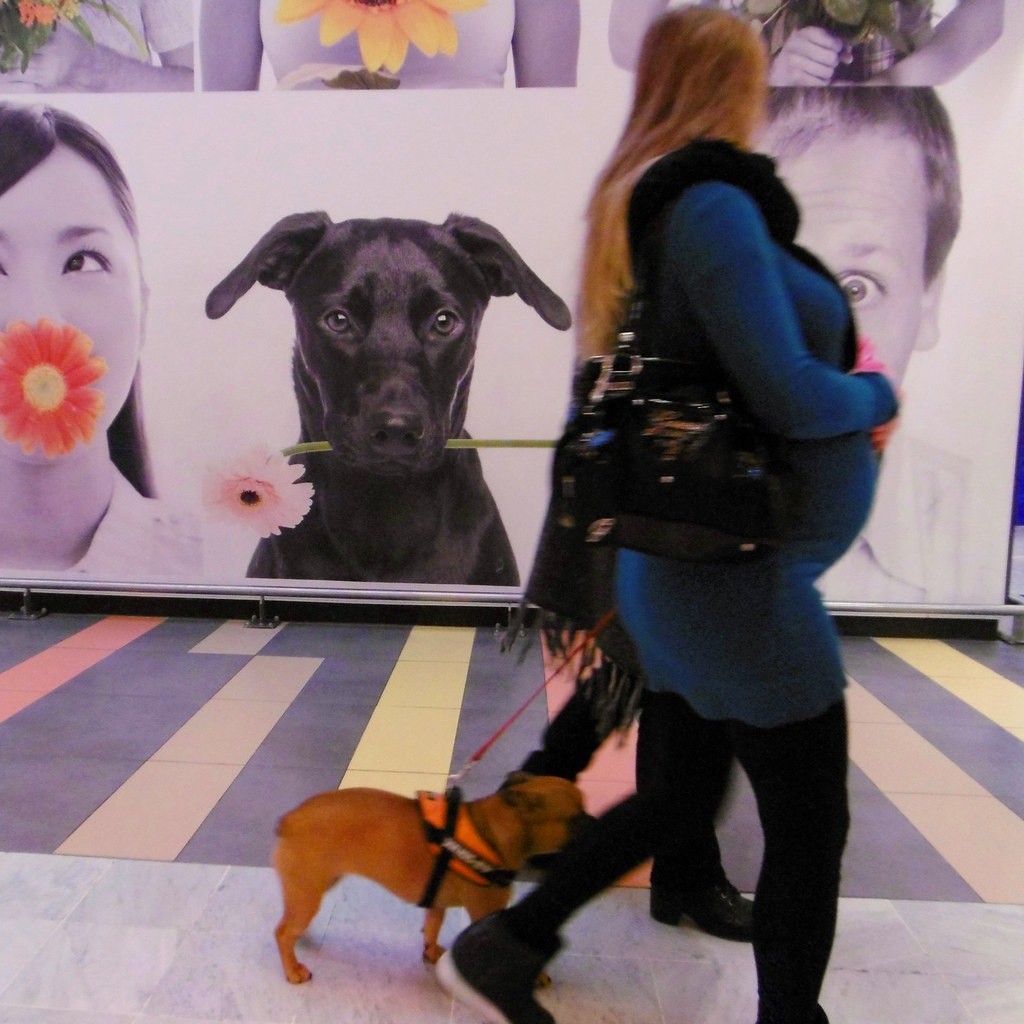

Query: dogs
[270,770,597,989]
[204,210,571,585]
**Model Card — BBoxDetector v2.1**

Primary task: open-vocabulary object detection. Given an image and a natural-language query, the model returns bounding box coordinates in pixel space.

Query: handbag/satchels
[570,181,776,569]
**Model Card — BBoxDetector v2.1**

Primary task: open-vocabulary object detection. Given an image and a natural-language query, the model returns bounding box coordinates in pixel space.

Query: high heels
[649,880,761,942]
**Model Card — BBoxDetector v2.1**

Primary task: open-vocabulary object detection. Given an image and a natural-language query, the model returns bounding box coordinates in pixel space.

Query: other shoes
[433,911,558,1023]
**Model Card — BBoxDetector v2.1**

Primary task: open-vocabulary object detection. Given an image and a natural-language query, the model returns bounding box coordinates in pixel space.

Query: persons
[200,0,579,92]
[757,87,1008,604]
[435,4,899,1024]
[1,101,218,578]
[607,0,1006,87]
[0,1,196,93]
